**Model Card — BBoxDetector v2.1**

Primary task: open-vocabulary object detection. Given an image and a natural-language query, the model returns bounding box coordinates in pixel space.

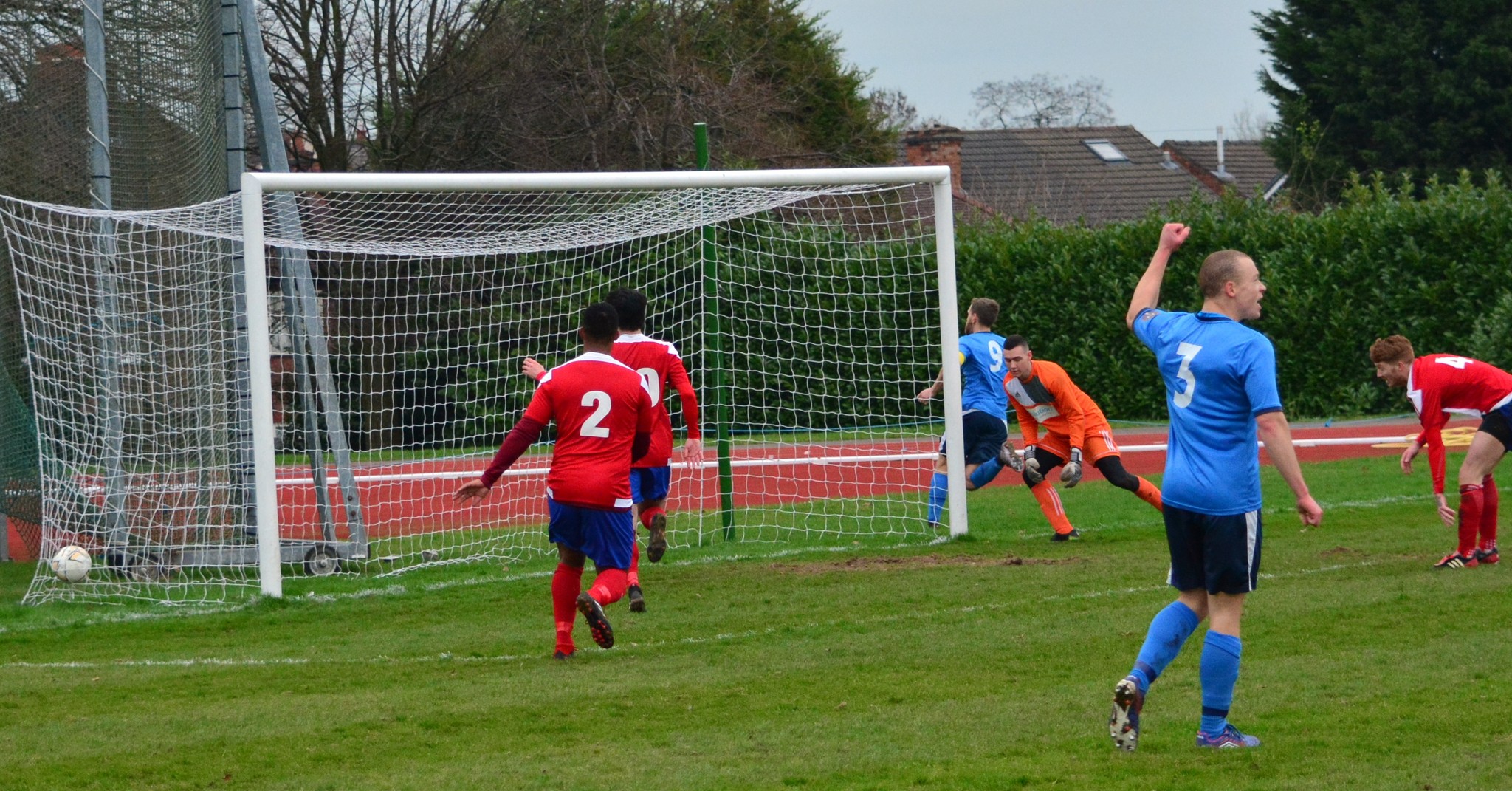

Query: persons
[452,303,652,659]
[917,298,1024,527]
[1370,334,1512,570]
[521,287,704,615]
[1002,335,1163,540]
[1109,222,1324,754]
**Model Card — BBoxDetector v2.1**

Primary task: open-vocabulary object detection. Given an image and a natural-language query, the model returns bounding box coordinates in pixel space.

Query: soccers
[50,545,93,584]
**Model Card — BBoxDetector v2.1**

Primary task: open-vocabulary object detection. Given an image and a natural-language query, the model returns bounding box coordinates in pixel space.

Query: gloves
[1060,452,1082,488]
[1022,450,1044,483]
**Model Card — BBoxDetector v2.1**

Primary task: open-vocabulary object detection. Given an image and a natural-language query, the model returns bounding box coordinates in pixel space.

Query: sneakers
[574,592,615,649]
[1001,441,1024,472]
[552,646,579,661]
[1050,529,1081,541]
[1434,549,1478,568]
[1473,546,1500,565]
[1109,675,1144,754]
[1196,724,1261,749]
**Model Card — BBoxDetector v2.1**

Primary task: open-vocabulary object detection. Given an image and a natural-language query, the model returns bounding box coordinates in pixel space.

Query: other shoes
[648,513,666,563]
[628,583,645,613]
